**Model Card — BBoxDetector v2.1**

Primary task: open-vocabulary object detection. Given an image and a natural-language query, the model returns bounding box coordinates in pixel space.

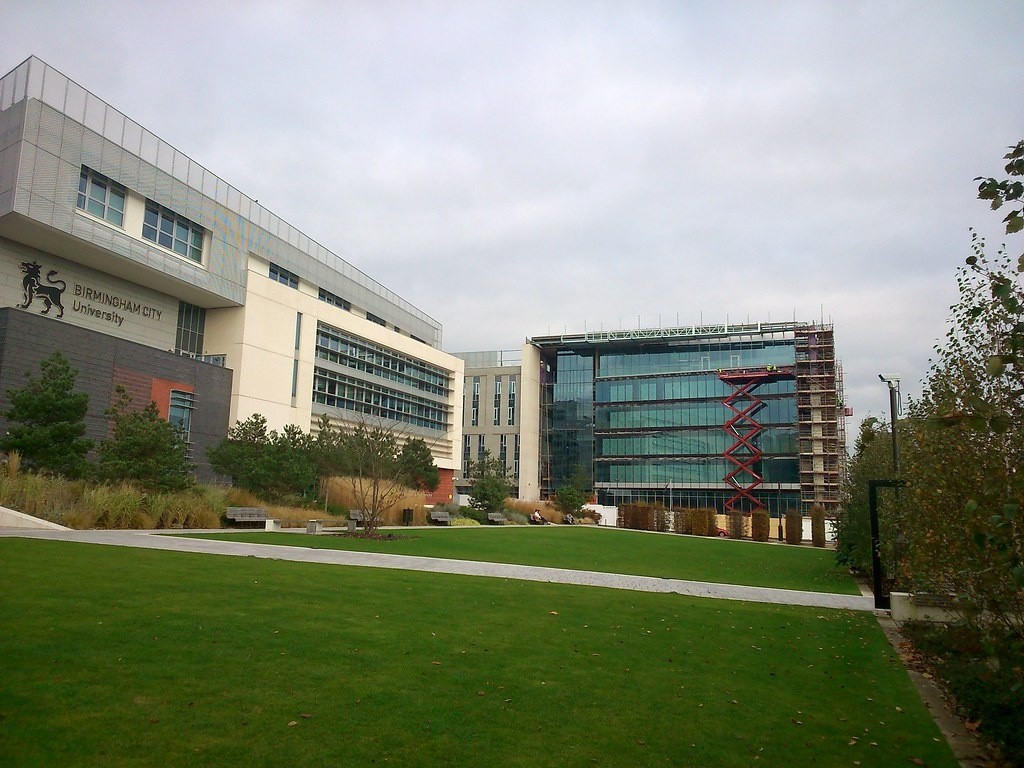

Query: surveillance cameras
[878,373,902,382]
[547,365,550,372]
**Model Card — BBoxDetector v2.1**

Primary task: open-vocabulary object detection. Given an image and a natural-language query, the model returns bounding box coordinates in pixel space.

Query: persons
[534,509,551,525]
[567,512,574,525]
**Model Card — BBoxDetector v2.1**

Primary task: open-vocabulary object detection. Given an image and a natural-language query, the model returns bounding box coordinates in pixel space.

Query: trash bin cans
[403,509,414,522]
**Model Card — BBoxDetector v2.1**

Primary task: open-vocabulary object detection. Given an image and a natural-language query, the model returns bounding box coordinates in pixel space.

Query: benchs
[431,511,455,525]
[349,510,384,526]
[487,512,507,525]
[226,506,285,528]
[563,515,568,523]
[529,514,540,524]
[303,521,358,535]
